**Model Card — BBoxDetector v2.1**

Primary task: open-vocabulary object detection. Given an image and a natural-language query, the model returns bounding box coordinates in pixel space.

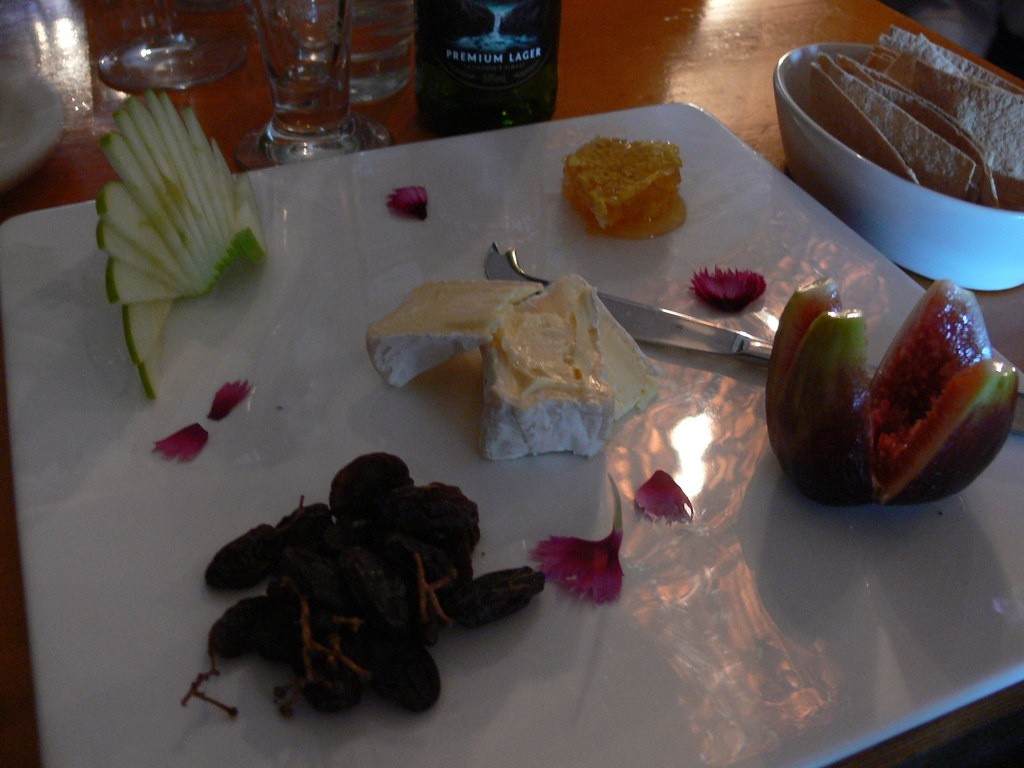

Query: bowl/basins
[773,42,1024,291]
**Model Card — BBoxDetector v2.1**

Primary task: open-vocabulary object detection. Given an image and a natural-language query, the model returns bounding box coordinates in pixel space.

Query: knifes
[486,244,772,370]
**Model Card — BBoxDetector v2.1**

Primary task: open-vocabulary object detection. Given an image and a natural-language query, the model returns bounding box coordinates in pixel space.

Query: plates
[0,67,69,190]
[0,100,1024,768]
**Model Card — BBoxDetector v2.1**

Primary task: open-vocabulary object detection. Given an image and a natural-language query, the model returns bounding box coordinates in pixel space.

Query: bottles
[415,0,561,135]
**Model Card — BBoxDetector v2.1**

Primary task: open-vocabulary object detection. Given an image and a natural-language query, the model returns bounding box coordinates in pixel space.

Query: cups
[343,0,416,103]
[230,0,389,170]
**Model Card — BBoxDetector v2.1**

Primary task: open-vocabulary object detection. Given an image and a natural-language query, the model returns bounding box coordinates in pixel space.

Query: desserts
[563,135,683,240]
[365,277,660,462]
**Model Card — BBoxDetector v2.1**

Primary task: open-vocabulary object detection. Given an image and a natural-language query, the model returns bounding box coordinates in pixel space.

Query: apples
[94,86,265,398]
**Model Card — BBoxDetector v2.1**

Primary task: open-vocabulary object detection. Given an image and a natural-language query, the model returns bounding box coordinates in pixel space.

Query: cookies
[809,22,1023,215]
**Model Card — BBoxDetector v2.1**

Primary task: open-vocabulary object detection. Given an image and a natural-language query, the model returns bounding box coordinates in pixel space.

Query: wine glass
[97,0,247,90]
